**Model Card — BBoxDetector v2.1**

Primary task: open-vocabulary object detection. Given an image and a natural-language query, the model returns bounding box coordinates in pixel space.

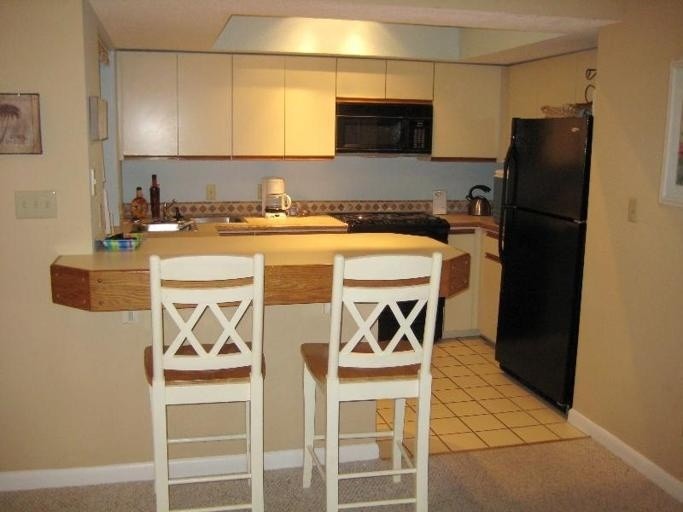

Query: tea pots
[465,184,491,216]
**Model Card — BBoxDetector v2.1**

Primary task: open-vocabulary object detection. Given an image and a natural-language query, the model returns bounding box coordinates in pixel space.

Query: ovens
[349,223,452,341]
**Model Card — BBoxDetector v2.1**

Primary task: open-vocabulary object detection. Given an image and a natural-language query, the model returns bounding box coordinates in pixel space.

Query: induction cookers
[330,211,449,227]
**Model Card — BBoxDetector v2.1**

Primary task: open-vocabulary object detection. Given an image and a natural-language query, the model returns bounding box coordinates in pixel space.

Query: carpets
[2,437,682,512]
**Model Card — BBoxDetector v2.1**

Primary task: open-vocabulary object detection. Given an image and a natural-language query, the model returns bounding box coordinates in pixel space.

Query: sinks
[190,216,248,224]
[130,223,190,232]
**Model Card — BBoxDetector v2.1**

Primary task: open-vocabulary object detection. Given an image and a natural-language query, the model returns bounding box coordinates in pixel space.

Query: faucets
[162,199,177,219]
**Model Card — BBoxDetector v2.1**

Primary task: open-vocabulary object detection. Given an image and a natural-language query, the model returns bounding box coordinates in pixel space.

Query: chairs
[301,253,442,512]
[145,253,263,512]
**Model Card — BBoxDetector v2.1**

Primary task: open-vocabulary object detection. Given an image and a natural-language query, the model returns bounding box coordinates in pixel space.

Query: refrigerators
[494,116,593,412]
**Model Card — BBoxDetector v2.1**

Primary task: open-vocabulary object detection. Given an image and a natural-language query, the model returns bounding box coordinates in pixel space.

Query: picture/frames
[659,58,683,206]
[0,93,43,155]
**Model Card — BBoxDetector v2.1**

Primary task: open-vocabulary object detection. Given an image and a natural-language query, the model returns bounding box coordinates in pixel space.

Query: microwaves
[336,103,434,153]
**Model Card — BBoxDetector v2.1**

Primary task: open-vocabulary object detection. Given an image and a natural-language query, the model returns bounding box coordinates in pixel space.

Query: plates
[101,232,144,250]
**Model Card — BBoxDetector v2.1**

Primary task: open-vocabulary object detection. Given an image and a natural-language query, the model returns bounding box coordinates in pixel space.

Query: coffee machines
[261,177,292,218]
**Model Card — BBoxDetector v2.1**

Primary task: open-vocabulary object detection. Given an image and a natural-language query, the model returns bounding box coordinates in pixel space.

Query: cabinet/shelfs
[480,229,505,345]
[233,56,336,158]
[433,63,502,158]
[118,48,232,159]
[337,56,433,102]
[442,227,480,340]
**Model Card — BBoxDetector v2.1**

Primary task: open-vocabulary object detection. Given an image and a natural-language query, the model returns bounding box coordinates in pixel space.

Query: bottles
[129,187,148,218]
[150,174,160,219]
